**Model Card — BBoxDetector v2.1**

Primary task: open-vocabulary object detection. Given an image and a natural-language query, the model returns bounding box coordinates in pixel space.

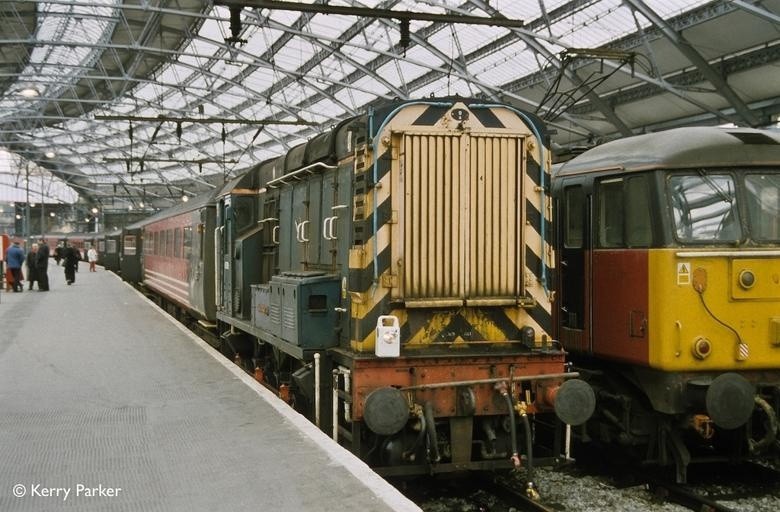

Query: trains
[543,124,780,485]
[11,93,597,489]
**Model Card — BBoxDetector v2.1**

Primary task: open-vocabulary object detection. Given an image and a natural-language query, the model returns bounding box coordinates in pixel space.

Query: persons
[5,239,26,292]
[26,242,40,290]
[62,242,77,286]
[72,243,81,273]
[36,237,51,293]
[53,242,62,266]
[87,244,98,272]
